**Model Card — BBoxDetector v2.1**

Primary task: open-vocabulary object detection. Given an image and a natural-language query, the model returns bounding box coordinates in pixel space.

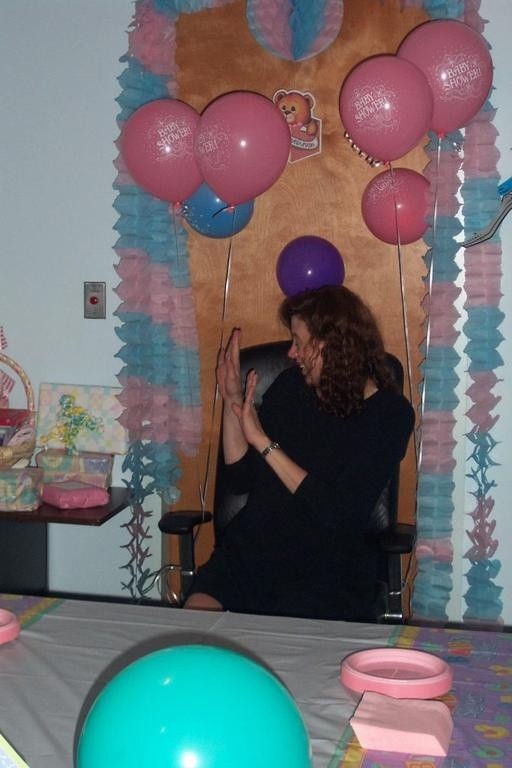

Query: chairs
[153,340,416,624]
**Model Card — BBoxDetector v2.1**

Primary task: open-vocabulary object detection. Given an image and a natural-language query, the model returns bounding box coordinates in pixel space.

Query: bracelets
[259,441,282,460]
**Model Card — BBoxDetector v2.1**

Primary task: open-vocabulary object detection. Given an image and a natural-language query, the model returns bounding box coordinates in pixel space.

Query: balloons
[122,97,205,208]
[398,18,494,138]
[338,52,437,161]
[76,642,311,768]
[362,166,434,243]
[276,236,345,294]
[181,180,256,238]
[194,90,292,210]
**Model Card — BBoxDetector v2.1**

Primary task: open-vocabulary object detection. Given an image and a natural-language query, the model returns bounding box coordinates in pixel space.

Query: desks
[1,592,511,768]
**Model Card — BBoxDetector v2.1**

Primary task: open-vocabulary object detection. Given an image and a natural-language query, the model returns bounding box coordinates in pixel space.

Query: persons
[182,286,417,624]
